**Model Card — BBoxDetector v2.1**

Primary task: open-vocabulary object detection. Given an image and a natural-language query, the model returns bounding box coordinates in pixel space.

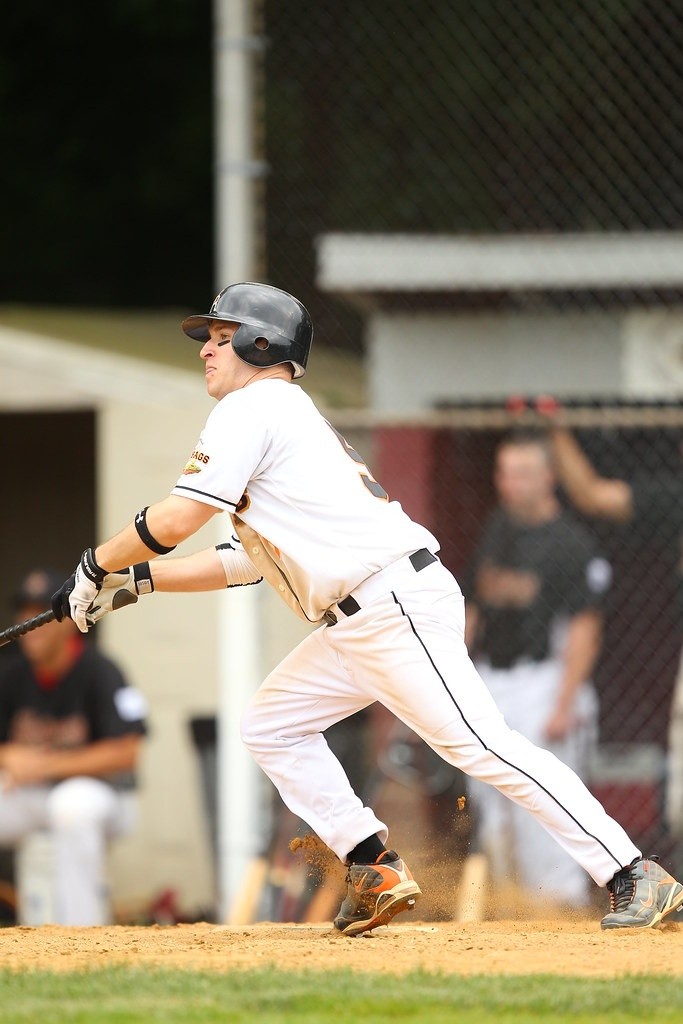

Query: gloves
[85,561,154,622]
[51,547,110,634]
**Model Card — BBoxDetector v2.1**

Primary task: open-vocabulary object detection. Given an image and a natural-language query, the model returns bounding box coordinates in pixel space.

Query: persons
[1,565,147,926]
[461,393,683,906]
[51,281,683,937]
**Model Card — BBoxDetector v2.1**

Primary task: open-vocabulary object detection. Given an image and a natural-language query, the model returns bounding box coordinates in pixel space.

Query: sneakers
[333,850,423,935]
[600,854,683,930]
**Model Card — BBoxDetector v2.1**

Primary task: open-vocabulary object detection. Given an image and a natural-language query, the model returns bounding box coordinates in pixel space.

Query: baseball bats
[0,607,59,647]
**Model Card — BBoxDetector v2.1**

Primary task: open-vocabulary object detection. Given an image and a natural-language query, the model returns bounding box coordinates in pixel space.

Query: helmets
[181,282,313,380]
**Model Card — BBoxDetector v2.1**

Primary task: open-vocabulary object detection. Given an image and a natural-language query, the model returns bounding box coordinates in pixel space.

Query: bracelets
[133,504,177,556]
